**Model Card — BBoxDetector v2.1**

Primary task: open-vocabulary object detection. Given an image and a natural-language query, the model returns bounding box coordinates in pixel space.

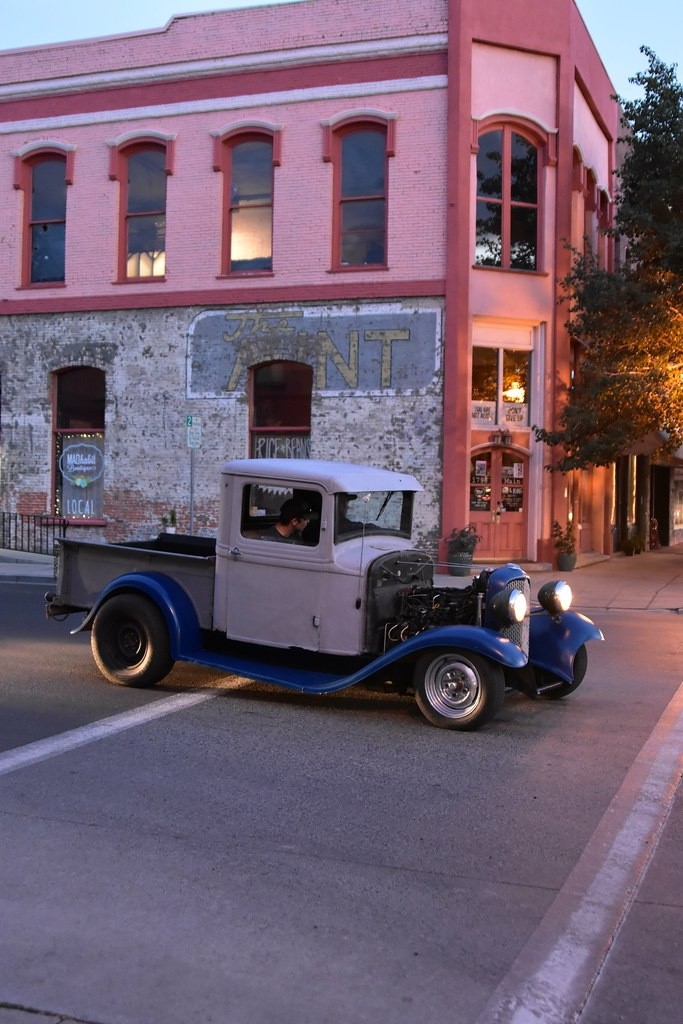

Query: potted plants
[444,525,483,576]
[550,520,577,572]
[620,535,646,557]
[161,510,176,534]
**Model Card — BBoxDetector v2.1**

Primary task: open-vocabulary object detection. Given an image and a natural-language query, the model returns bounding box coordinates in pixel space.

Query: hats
[335,491,357,503]
[280,498,318,520]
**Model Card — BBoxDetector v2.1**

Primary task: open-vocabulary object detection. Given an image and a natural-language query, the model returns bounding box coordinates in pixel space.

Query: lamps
[502,428,512,446]
[494,429,502,446]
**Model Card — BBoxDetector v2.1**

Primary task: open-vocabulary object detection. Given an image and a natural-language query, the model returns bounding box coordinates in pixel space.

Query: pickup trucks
[43,458,605,732]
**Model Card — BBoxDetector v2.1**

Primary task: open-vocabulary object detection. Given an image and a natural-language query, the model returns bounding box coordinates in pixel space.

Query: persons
[301,493,383,543]
[259,500,317,545]
